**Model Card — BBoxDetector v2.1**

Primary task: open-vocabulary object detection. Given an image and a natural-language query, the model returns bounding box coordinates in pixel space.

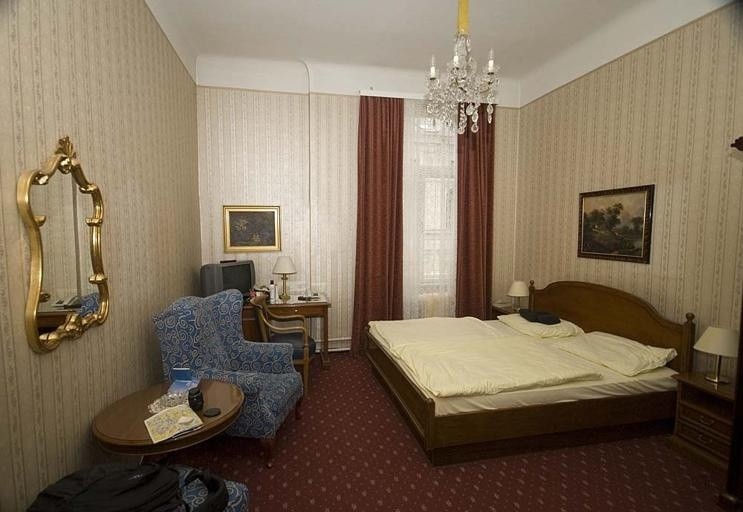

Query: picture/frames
[577,184,658,264]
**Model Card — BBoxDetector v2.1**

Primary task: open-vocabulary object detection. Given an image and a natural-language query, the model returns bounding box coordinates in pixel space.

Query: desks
[36,298,79,328]
[91,381,245,466]
[240,297,333,369]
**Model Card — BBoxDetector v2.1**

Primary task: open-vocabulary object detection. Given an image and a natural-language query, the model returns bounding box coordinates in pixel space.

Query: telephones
[491,299,512,308]
[63,296,82,309]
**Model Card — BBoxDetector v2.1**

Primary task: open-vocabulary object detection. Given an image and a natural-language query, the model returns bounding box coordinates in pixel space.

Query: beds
[362,280,696,466]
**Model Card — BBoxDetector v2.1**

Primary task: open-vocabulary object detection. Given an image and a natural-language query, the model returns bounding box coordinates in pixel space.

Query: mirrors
[13,135,110,354]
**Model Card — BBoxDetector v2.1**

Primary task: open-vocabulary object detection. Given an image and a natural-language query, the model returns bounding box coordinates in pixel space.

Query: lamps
[222,204,282,251]
[271,256,296,298]
[507,280,529,310]
[422,1,501,137]
[692,325,740,385]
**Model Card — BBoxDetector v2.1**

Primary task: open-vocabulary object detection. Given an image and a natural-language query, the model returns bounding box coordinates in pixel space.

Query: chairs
[249,296,316,391]
[150,287,303,454]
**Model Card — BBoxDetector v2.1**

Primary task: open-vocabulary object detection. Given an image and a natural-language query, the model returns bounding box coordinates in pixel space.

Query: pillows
[497,310,586,337]
[557,329,678,378]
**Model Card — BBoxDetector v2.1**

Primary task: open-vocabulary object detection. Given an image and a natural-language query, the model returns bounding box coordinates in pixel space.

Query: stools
[30,462,249,512]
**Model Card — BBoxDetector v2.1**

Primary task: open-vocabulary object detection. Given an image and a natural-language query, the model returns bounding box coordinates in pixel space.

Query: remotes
[220,260,236,263]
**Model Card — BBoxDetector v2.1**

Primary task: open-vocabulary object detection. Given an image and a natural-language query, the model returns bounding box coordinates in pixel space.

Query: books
[142,404,203,444]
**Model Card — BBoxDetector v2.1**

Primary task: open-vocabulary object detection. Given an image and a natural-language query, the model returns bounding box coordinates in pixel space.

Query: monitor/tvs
[200,261,255,298]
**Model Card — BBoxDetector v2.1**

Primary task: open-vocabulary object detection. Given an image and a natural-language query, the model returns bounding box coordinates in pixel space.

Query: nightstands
[492,303,527,320]
[671,373,736,461]
